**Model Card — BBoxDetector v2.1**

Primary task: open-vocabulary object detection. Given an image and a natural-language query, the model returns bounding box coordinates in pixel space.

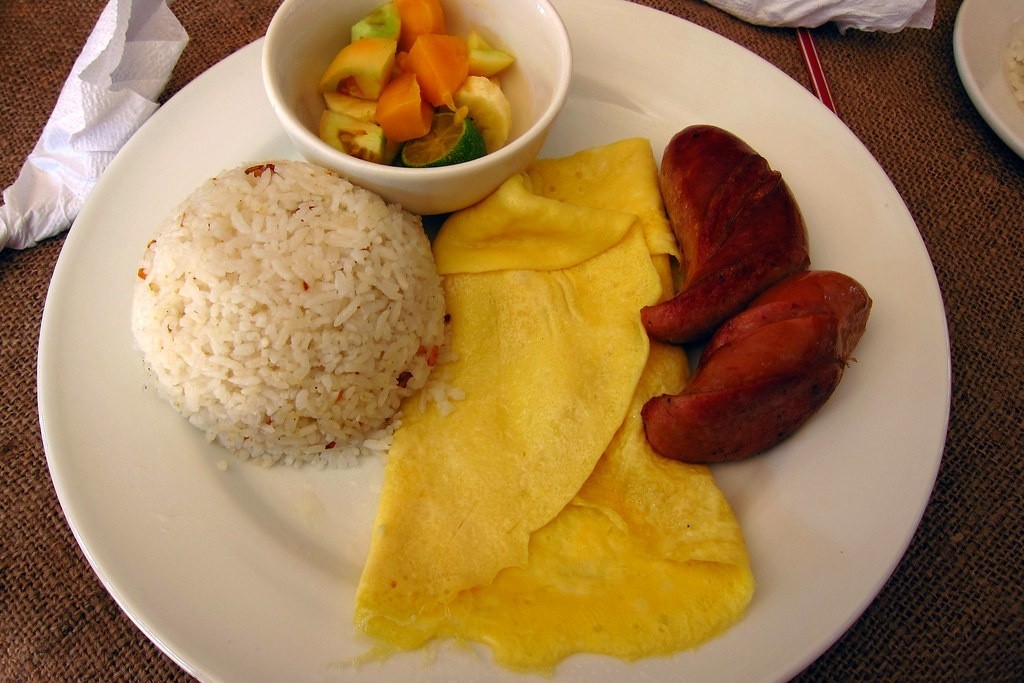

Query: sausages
[632,123,876,464]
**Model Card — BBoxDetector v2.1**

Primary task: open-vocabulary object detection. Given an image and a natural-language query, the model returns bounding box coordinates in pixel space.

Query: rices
[132,157,452,475]
[1003,10,1021,108]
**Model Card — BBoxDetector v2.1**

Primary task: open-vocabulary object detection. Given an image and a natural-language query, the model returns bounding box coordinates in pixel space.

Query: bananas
[317,0,520,176]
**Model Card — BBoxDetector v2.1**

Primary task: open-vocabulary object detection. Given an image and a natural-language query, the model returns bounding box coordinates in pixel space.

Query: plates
[34,0,953,683]
[952,0,1024,159]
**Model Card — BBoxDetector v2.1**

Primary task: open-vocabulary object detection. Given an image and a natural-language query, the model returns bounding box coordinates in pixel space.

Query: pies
[323,132,758,668]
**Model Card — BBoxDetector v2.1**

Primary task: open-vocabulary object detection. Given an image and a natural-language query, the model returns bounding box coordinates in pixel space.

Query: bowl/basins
[264,0,575,212]
